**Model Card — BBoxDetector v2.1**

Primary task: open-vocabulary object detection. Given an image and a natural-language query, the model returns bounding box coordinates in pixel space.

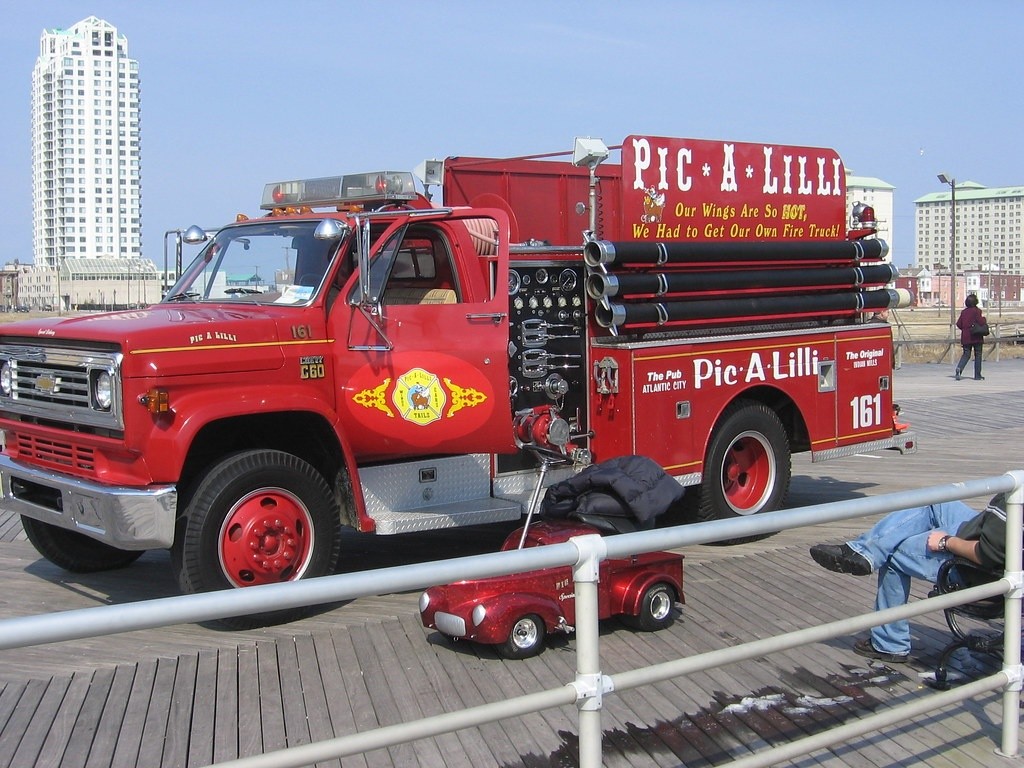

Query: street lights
[938,173,957,363]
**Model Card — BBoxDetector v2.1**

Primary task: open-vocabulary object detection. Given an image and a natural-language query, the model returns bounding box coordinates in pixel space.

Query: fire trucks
[0,134,923,631]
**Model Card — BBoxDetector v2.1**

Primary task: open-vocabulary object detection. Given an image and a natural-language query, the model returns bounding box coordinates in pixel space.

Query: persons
[811,493,1006,663]
[955,294,986,380]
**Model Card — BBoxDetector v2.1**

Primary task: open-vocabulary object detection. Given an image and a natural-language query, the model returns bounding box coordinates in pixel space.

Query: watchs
[938,535,952,553]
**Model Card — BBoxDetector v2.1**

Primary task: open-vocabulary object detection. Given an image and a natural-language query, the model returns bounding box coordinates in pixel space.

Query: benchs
[328,289,457,305]
[927,558,1004,690]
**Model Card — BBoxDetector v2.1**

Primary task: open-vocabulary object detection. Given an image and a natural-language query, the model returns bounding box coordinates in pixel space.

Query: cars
[418,518,685,662]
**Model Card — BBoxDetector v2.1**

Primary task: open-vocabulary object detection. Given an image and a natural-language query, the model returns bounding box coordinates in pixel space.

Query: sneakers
[810,544,871,577]
[854,639,908,663]
[974,375,985,380]
[956,367,962,380]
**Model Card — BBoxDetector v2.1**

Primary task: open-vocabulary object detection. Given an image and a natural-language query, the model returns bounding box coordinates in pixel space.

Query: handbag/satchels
[971,322,990,336]
